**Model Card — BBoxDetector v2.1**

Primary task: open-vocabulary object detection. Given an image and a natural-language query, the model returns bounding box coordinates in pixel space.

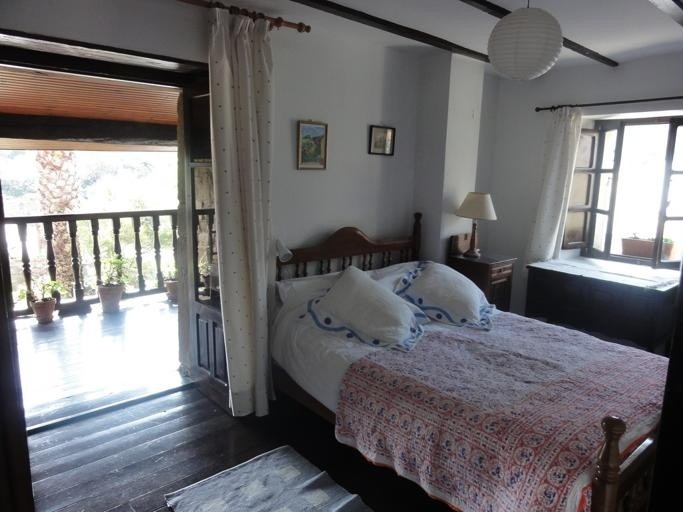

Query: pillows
[312,257,496,353]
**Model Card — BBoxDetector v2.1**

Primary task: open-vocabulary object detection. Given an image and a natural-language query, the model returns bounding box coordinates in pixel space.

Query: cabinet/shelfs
[525,261,681,362]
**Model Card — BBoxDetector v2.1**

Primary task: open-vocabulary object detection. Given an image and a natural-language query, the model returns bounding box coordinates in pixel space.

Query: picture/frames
[369,124,396,156]
[297,119,328,169]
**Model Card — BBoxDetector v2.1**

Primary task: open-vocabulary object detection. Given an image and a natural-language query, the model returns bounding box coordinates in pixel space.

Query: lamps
[275,237,294,263]
[487,0,563,82]
[455,191,499,259]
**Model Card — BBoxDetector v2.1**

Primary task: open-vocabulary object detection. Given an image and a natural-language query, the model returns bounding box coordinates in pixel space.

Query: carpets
[164,443,368,512]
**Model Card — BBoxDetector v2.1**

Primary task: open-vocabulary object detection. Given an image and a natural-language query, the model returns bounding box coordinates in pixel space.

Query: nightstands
[448,253,518,312]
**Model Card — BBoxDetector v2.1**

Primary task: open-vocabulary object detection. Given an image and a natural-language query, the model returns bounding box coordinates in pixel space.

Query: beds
[273,211,682,511]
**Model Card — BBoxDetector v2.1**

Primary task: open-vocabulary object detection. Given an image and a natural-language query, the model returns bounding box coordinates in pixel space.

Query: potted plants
[98,256,135,311]
[164,271,181,305]
[620,233,673,260]
[15,281,74,325]
